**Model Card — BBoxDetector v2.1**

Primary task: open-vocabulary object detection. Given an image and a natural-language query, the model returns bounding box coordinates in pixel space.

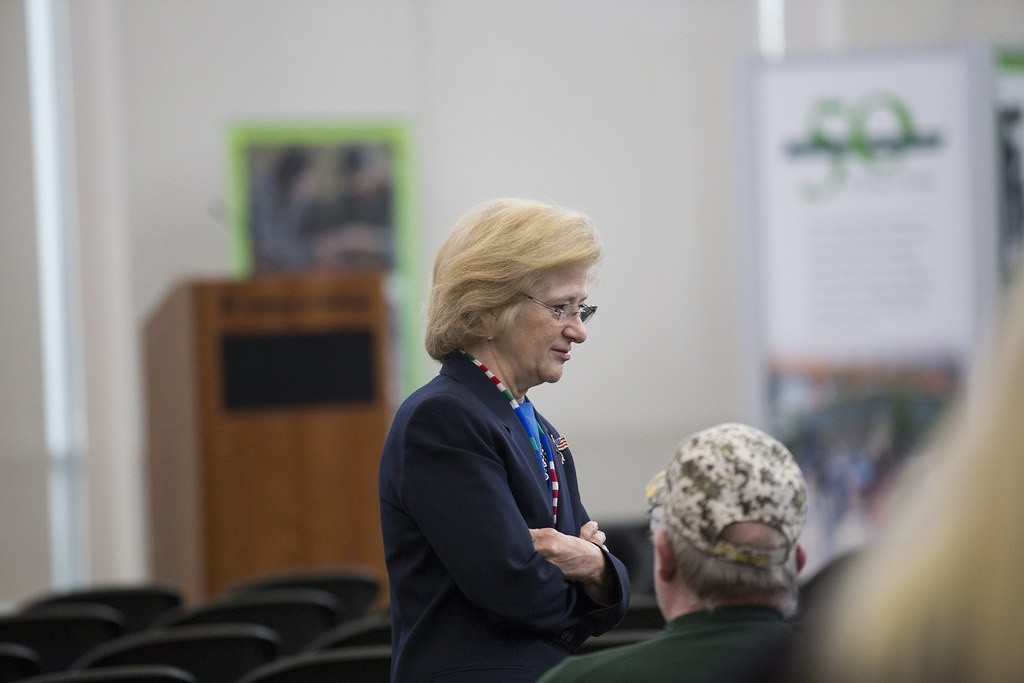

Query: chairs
[0,571,393,683]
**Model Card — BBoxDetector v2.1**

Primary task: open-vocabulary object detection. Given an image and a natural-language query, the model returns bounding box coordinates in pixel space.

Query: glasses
[523,292,597,325]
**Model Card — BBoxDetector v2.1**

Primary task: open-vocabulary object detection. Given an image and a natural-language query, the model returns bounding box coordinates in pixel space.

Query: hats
[647,423,810,566]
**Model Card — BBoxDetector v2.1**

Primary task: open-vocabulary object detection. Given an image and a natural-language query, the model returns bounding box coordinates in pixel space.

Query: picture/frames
[228,123,420,403]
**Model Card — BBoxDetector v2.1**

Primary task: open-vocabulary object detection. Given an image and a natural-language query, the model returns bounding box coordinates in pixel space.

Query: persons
[379,198,630,683]
[536,300,1024,683]
